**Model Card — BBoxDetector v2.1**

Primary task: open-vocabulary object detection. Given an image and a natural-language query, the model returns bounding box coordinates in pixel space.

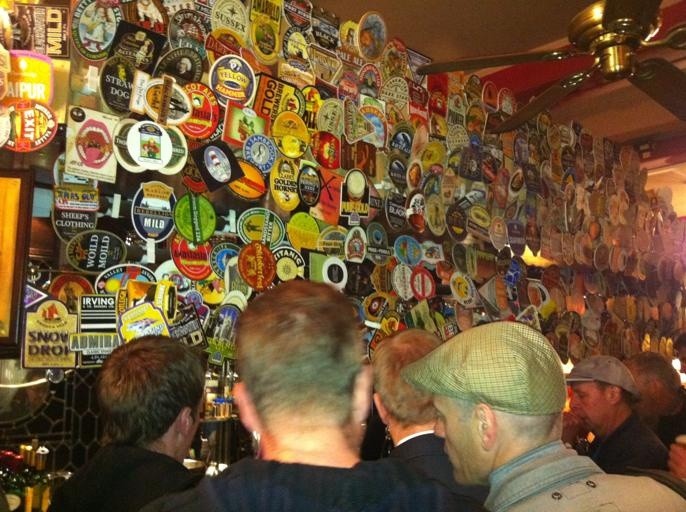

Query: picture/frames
[0,165,37,360]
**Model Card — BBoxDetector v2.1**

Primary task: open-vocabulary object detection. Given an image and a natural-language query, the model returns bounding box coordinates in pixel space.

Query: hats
[563,353,645,405]
[396,319,570,419]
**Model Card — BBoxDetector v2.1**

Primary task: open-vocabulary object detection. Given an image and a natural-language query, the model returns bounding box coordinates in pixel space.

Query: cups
[347,170,366,203]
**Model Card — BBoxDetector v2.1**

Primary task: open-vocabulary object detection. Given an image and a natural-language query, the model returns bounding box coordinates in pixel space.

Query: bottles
[0,444,56,512]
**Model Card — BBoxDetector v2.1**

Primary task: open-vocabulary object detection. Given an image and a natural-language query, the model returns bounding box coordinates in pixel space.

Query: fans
[415,1,686,135]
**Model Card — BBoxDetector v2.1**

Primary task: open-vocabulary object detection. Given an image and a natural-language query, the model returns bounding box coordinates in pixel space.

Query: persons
[669,331,686,485]
[45,335,210,512]
[398,321,685,512]
[374,327,491,506]
[563,354,668,475]
[138,279,491,512]
[620,351,686,451]
